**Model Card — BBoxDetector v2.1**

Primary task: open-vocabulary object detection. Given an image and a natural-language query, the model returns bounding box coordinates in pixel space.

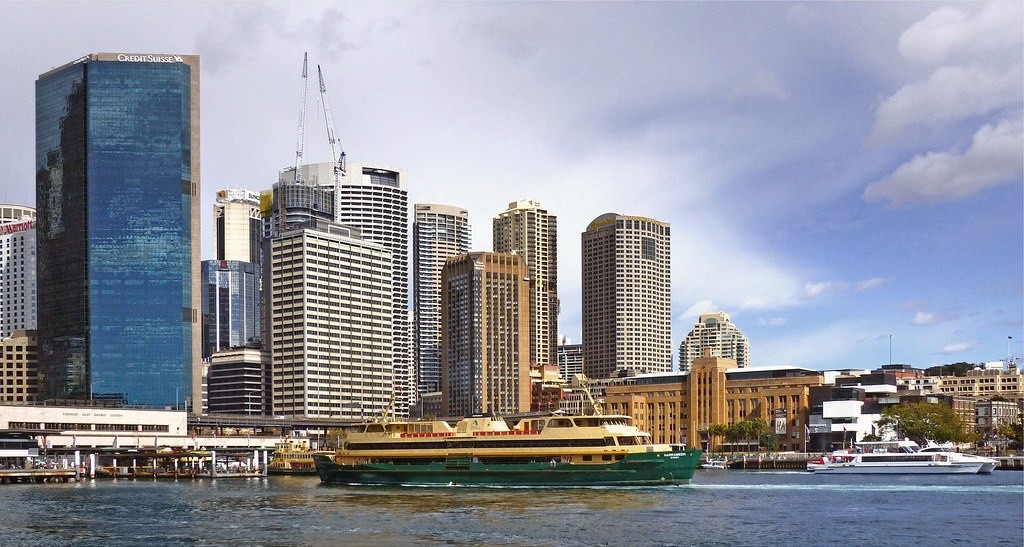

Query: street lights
[888,334,893,366]
[90,380,104,407]
[249,392,254,416]
[175,386,188,410]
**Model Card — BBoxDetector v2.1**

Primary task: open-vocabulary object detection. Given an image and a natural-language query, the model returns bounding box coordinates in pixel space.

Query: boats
[701,456,730,469]
[311,372,703,490]
[807,428,1000,473]
[267,432,335,476]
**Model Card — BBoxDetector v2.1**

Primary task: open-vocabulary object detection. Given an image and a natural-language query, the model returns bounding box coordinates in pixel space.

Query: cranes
[318,64,348,222]
[294,51,308,182]
